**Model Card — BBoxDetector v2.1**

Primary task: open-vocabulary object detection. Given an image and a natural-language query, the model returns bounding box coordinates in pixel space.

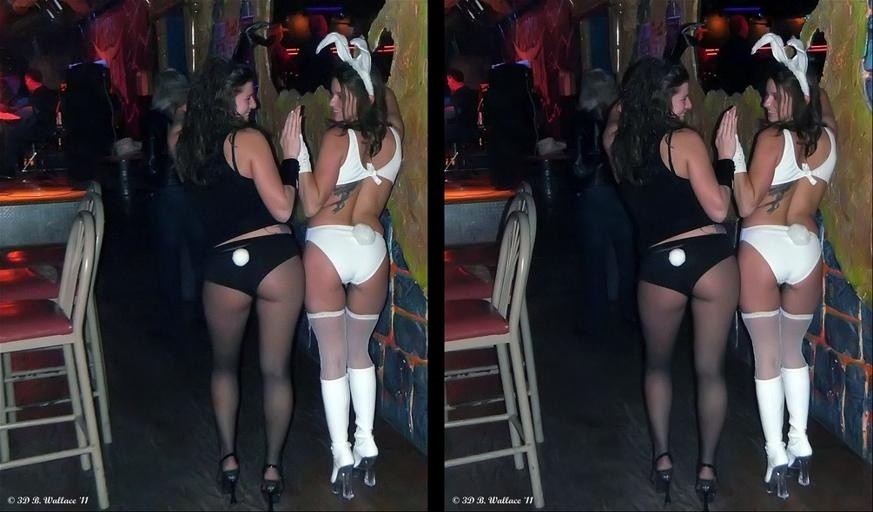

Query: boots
[348,364,379,488]
[321,374,354,499]
[781,364,812,486]
[755,374,790,500]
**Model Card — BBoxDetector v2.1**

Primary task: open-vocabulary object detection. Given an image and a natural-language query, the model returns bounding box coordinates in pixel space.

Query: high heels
[261,464,284,512]
[695,463,717,512]
[217,452,240,505]
[651,451,673,504]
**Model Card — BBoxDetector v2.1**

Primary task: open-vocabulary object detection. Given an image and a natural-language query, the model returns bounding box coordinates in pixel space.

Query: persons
[146,66,208,338]
[601,54,740,512]
[0,68,58,172]
[295,31,407,499]
[567,66,637,335]
[446,69,478,126]
[172,53,311,510]
[729,32,838,501]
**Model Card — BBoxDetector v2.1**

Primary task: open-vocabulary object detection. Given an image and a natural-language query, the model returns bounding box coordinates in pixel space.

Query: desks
[443,180,535,265]
[2,180,111,270]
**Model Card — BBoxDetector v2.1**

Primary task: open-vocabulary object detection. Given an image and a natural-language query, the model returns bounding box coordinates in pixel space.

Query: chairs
[437,190,548,449]
[443,206,547,512]
[2,207,115,511]
[1,195,118,451]
